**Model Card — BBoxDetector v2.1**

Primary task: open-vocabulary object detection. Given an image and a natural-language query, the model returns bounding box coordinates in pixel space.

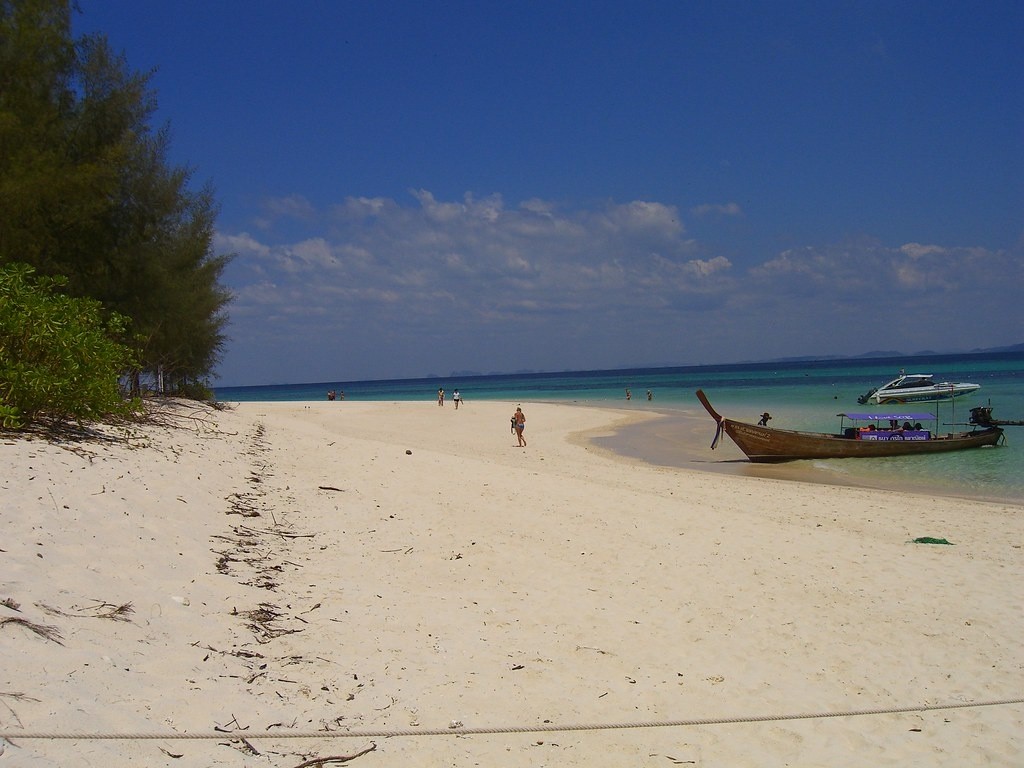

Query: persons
[888,419,903,433]
[453,389,462,409]
[903,422,915,432]
[327,390,345,400]
[513,407,526,447]
[915,423,924,430]
[758,412,772,426]
[439,387,444,406]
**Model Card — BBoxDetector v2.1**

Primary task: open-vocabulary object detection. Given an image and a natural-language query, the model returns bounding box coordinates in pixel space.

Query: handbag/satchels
[511,418,516,435]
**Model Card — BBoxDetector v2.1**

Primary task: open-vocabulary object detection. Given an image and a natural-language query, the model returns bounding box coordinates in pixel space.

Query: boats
[856,367,980,405]
[693,389,1023,464]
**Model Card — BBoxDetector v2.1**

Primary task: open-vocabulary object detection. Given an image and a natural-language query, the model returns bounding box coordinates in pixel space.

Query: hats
[760,413,772,420]
